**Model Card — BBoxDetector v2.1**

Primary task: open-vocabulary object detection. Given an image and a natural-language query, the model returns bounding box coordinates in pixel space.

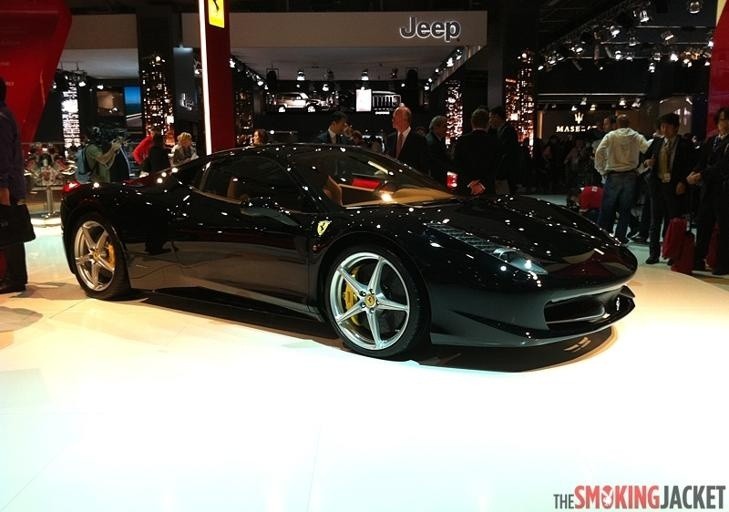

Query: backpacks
[75,142,100,185]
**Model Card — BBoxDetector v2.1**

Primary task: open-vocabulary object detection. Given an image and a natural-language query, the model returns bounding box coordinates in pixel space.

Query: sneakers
[621,222,659,264]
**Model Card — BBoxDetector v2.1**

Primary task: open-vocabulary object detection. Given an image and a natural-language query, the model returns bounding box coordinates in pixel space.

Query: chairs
[224,176,247,201]
[319,174,344,205]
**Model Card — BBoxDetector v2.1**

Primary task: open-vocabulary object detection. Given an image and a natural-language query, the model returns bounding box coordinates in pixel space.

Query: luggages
[662,184,694,275]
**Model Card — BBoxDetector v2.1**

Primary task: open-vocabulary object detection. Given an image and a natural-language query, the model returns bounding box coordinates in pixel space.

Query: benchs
[30,184,64,218]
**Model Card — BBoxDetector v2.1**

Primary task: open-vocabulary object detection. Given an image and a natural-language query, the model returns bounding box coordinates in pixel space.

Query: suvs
[271,91,330,114]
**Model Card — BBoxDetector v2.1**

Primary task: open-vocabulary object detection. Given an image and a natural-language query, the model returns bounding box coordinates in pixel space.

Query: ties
[396,133,402,159]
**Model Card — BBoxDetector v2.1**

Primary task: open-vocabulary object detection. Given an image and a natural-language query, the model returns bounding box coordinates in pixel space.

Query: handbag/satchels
[0,204,35,247]
[143,157,151,172]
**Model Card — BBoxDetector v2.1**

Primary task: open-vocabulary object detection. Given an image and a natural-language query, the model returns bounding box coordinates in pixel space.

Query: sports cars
[56,142,639,359]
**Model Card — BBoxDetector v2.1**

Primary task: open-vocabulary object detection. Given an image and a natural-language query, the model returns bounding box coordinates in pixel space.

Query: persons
[253,128,268,146]
[602,115,646,236]
[594,114,654,244]
[21,124,200,194]
[643,113,690,265]
[0,78,36,294]
[685,108,729,275]
[313,101,706,195]
